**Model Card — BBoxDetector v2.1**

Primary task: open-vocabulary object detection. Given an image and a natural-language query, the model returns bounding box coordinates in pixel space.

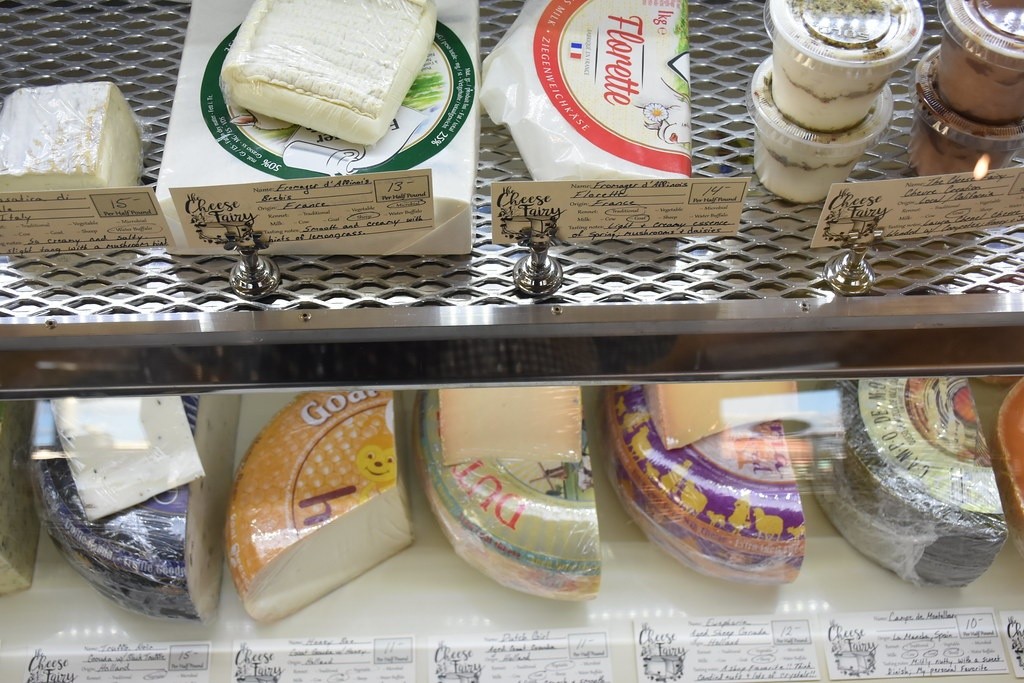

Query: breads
[0,375,1024,622]
[0,0,693,189]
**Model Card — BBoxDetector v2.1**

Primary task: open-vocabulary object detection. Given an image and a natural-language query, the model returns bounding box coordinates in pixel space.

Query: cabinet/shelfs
[0,0,1024,683]
